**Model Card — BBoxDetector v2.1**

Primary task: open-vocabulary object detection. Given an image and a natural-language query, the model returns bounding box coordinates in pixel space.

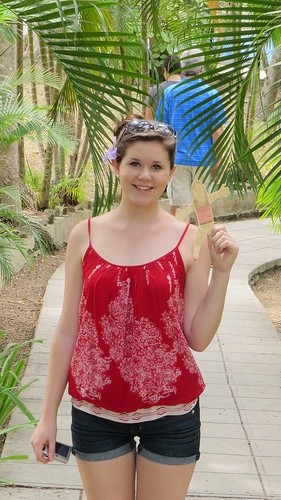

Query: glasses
[114,119,177,158]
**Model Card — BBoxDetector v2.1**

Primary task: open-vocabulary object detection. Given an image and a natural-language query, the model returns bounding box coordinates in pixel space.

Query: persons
[156,48,229,220]
[30,113,241,499]
[143,54,183,122]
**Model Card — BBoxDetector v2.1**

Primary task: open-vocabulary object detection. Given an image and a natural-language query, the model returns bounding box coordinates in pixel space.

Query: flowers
[102,145,119,163]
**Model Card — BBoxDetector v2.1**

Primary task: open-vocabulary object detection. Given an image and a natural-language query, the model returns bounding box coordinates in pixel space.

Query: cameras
[43,442,71,463]
